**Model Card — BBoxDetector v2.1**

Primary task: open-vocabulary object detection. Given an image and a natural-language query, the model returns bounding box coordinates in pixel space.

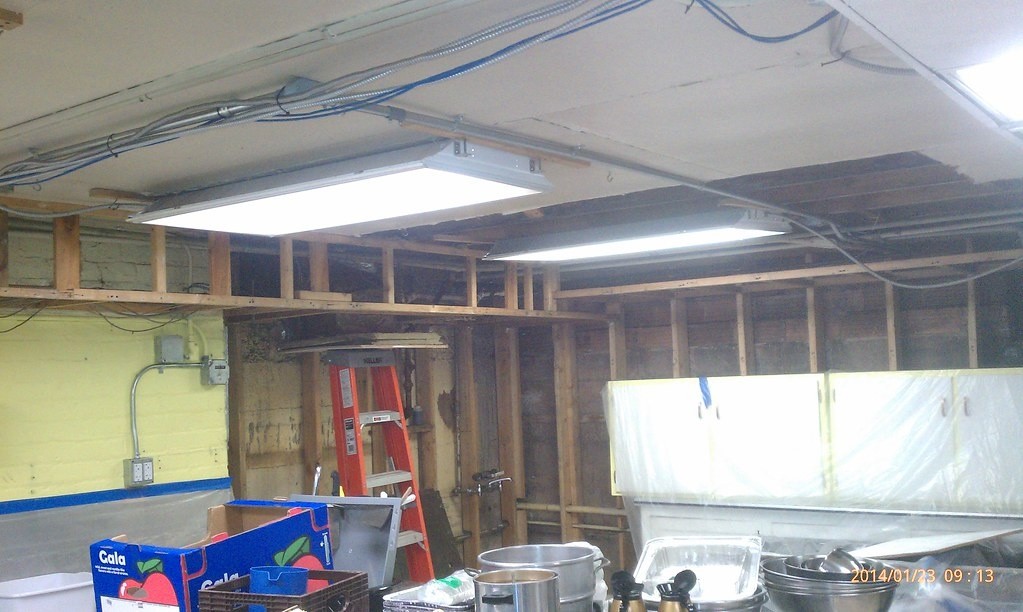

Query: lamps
[123,137,544,236]
[482,216,796,262]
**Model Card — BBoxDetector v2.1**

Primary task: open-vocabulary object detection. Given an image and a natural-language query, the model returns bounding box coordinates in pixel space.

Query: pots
[464,542,611,612]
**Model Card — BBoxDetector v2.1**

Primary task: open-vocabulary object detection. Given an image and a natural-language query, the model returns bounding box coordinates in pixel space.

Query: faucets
[488,477,513,493]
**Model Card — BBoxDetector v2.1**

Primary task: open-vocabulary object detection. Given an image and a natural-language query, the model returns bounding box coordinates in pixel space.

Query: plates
[633,534,762,600]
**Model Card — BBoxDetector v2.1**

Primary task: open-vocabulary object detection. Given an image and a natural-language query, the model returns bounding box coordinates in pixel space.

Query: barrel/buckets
[247,565,309,612]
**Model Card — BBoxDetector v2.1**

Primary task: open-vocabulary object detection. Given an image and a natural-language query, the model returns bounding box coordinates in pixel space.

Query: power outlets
[123,456,154,487]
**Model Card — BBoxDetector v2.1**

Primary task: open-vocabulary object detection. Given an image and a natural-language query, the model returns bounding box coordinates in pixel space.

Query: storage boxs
[0,569,96,612]
[89,499,334,612]
[383,568,475,612]
[197,566,372,611]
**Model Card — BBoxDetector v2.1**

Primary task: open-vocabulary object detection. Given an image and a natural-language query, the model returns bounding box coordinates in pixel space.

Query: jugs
[609,569,697,612]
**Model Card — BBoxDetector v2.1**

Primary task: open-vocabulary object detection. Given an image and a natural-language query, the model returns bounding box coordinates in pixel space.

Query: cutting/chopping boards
[843,528,1022,561]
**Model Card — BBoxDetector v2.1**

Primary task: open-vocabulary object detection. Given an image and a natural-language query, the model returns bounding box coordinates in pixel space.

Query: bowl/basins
[630,584,769,612]
[760,548,900,612]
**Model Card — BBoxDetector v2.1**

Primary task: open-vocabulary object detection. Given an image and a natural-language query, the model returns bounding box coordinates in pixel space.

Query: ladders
[329,350,435,585]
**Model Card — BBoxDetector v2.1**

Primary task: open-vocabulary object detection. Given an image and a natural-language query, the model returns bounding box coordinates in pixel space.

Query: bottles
[413,401,423,426]
